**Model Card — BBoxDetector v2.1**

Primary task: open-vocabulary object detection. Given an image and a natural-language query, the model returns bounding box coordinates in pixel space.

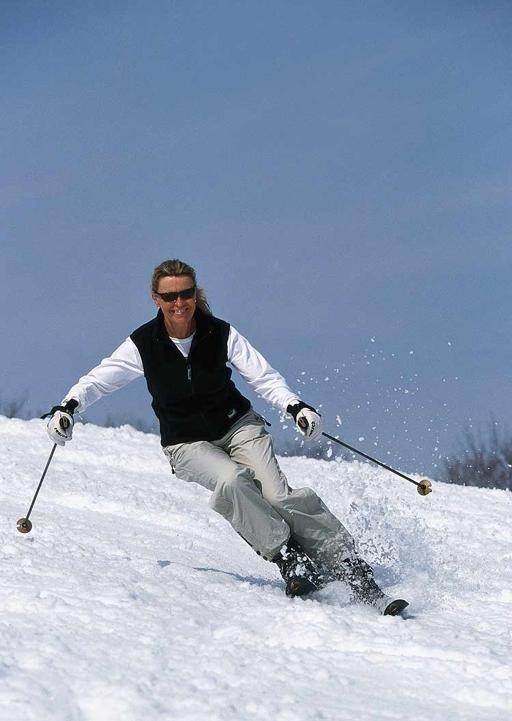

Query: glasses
[153,283,198,302]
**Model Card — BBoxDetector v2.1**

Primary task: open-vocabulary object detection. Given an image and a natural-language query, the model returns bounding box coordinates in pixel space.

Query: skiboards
[286,562,408,616]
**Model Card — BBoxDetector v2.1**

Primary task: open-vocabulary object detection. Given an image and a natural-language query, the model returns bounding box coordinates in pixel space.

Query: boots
[271,535,327,599]
[329,553,409,617]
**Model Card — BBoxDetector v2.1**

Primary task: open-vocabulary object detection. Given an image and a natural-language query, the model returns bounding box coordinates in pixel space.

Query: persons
[48,258,380,599]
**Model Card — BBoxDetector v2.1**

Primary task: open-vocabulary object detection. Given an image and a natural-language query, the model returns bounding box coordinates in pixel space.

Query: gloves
[287,400,323,441]
[40,397,81,447]
